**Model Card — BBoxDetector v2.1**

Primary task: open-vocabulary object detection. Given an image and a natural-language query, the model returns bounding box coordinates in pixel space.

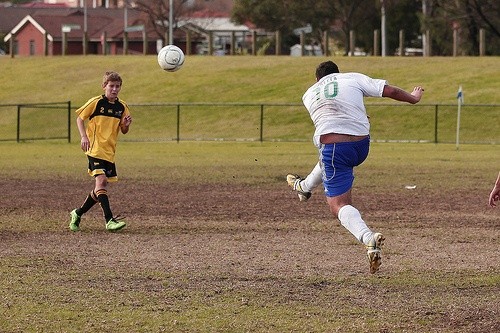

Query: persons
[489,172,500,208]
[69,71,132,231]
[287,60,424,274]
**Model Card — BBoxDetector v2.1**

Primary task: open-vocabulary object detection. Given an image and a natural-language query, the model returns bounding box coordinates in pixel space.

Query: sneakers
[70,209,81,231]
[287,174,311,202]
[366,233,385,274]
[106,215,127,230]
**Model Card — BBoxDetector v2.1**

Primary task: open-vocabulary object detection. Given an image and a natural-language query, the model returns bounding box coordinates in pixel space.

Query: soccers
[157,45,185,73]
